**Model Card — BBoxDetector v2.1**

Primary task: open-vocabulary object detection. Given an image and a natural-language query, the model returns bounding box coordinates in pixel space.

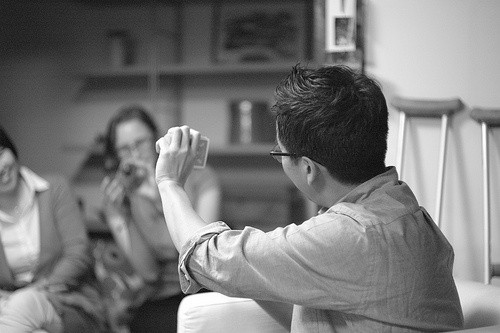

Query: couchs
[175,279,500,333]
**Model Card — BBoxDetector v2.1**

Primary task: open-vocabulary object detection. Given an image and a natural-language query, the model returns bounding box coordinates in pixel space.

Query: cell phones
[180,135,210,169]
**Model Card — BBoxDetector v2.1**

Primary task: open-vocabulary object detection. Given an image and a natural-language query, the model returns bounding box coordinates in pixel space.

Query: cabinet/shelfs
[83,63,307,227]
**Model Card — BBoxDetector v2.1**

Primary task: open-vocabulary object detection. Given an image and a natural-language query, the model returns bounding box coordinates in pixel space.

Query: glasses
[271,142,323,166]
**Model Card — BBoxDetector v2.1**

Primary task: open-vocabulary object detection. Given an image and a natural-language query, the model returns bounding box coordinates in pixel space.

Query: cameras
[116,164,147,194]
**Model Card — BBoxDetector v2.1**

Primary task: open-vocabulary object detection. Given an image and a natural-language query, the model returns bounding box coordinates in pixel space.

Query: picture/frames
[210,1,313,63]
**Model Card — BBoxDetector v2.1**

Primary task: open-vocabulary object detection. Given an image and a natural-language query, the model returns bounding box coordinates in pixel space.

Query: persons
[96,105,221,333]
[0,126,112,333]
[152,63,465,332]
